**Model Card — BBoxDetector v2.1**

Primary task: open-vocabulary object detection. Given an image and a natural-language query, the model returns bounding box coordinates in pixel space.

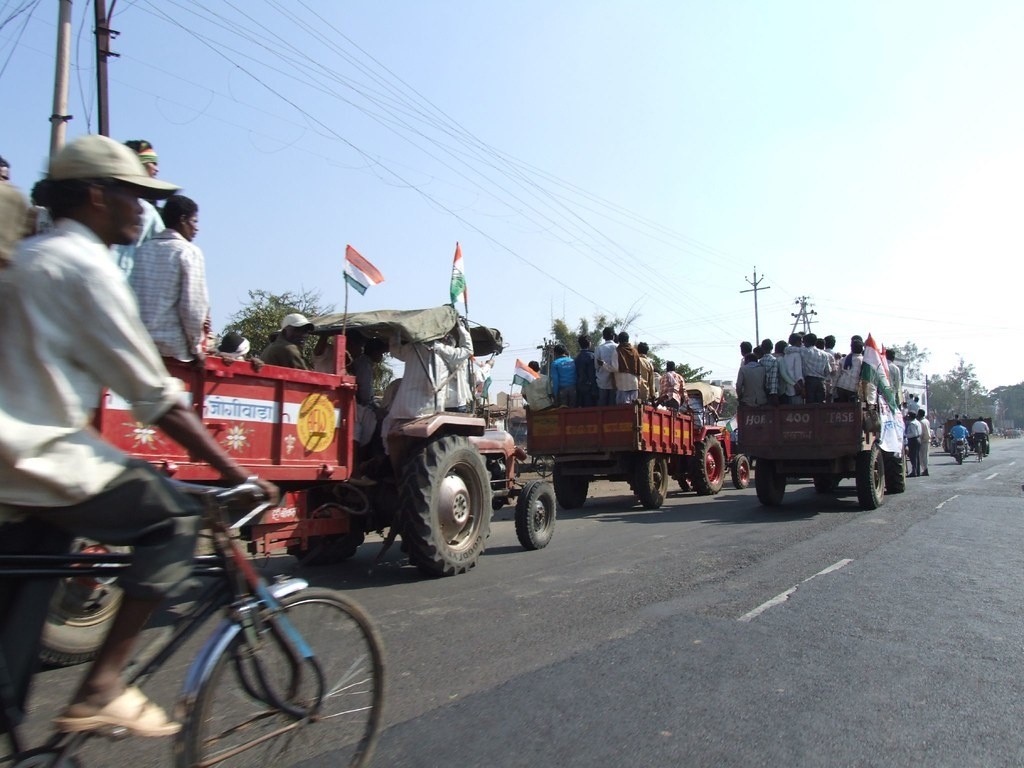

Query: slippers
[51,686,184,738]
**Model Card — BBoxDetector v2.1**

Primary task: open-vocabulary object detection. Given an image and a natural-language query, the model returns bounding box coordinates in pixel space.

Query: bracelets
[244,473,259,484]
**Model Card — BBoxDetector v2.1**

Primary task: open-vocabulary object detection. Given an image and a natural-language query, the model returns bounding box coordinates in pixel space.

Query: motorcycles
[948,434,969,463]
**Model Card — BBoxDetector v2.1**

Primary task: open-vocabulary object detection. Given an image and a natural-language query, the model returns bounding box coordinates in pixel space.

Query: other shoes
[905,473,921,478]
[921,473,930,476]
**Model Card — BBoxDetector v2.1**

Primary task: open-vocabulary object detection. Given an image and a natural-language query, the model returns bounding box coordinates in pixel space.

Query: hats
[281,314,314,333]
[50,134,183,201]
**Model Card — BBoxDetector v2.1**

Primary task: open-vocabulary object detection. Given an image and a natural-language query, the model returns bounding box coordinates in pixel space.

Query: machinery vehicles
[942,417,994,454]
[525,381,750,494]
[20,307,558,666]
[736,393,907,510]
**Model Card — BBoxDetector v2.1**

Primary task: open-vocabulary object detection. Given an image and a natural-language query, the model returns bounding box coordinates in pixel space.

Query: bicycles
[510,449,554,478]
[0,480,388,767]
[973,434,987,462]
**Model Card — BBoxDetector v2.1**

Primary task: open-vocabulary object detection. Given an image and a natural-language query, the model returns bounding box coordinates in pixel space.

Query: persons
[1004,424,1022,439]
[972,415,990,457]
[520,361,554,413]
[549,326,694,415]
[0,135,318,739]
[930,426,944,447]
[311,304,474,487]
[952,413,969,420]
[736,331,902,409]
[901,393,920,417]
[904,408,931,478]
[948,421,970,456]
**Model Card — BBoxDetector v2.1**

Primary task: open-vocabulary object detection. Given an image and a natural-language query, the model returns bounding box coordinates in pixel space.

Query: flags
[513,360,541,388]
[344,244,384,297]
[861,333,897,415]
[726,412,739,434]
[880,345,894,390]
[450,245,467,304]
